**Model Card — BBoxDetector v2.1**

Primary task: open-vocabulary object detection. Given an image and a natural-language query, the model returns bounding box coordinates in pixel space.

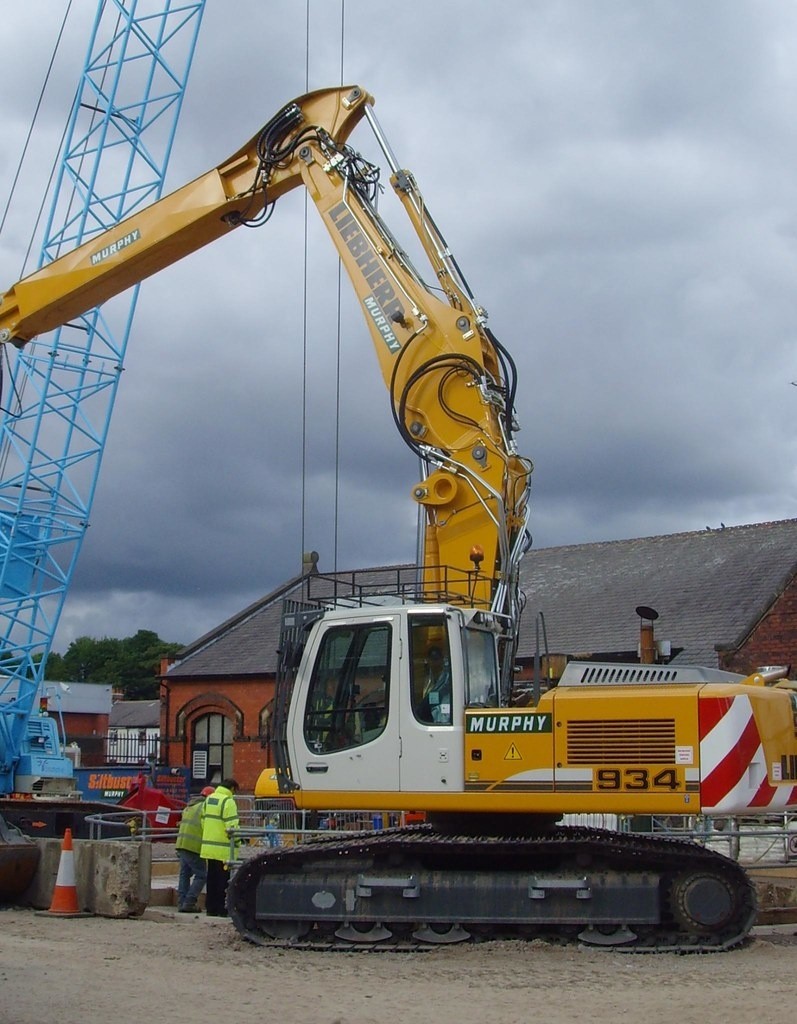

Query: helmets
[200,786,216,796]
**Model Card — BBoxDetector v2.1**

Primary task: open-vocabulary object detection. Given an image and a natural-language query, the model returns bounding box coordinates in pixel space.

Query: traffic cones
[33,826,96,918]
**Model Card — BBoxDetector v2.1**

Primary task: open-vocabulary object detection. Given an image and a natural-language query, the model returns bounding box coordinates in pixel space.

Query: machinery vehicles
[0,82,796,953]
[0,0,209,911]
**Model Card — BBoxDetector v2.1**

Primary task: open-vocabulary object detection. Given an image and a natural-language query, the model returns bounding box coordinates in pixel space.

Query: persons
[201,778,240,918]
[412,645,445,707]
[173,786,215,913]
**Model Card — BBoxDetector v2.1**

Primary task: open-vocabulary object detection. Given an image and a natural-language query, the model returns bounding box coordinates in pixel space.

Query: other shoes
[176,902,203,913]
[206,907,229,917]
